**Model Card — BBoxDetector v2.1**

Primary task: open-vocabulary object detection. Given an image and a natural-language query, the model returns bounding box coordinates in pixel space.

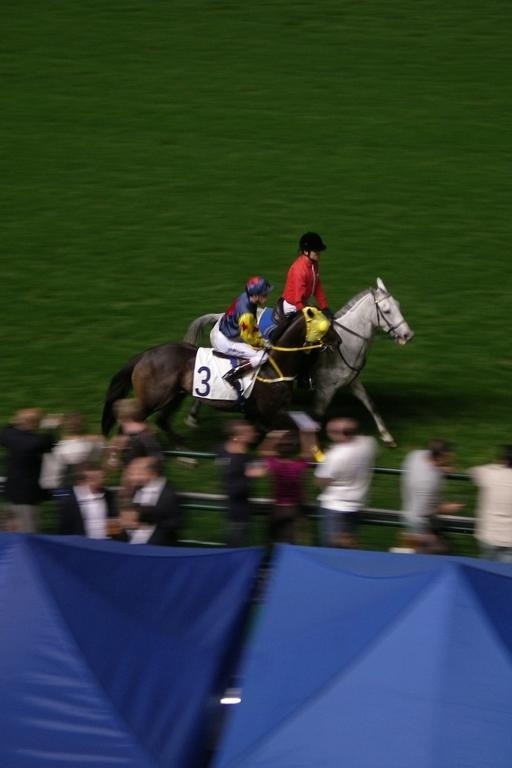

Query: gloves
[320,307,335,319]
[260,337,272,350]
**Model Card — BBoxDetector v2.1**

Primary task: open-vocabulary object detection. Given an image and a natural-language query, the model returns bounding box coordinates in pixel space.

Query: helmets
[246,275,275,296]
[299,232,327,252]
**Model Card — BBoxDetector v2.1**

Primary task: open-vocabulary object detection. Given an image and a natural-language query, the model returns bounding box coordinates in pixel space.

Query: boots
[222,361,251,392]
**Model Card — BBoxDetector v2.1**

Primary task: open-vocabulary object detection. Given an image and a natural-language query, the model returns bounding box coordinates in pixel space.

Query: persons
[399,438,464,555]
[468,444,512,562]
[268,232,335,340]
[209,276,275,392]
[221,418,318,547]
[314,420,377,549]
[1,409,182,545]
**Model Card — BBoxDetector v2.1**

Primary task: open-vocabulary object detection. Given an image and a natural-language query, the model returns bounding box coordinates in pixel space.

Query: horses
[101,294,342,469]
[183,278,415,448]
